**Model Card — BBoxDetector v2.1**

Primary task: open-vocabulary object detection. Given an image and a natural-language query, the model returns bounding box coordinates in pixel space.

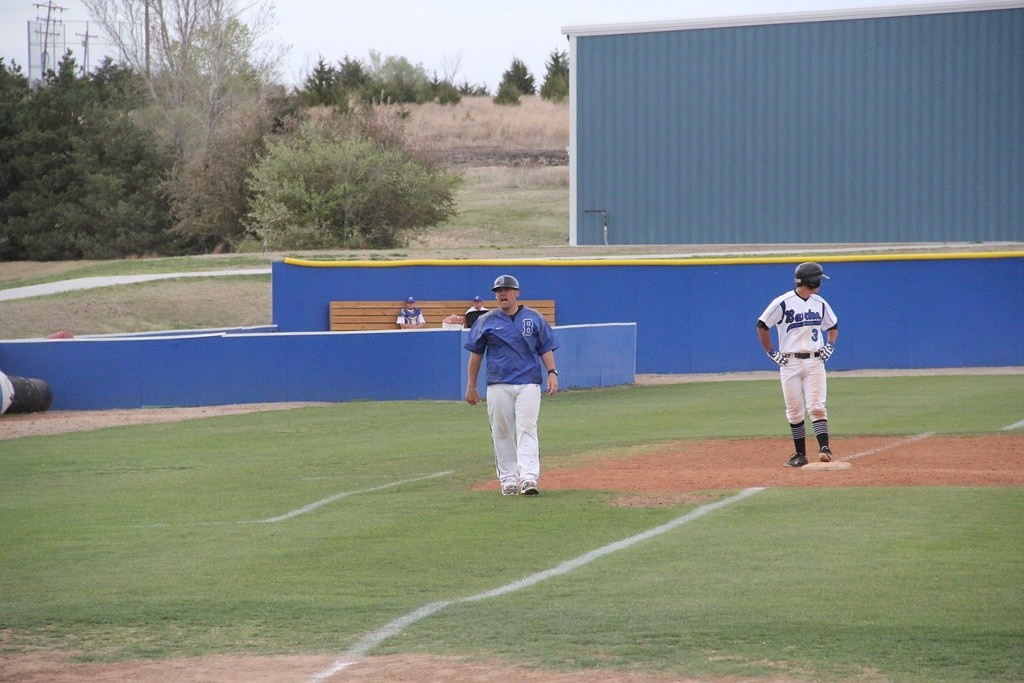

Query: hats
[405,296,415,303]
[473,295,483,302]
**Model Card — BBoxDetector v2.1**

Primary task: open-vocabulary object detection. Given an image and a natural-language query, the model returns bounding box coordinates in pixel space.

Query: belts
[795,353,820,359]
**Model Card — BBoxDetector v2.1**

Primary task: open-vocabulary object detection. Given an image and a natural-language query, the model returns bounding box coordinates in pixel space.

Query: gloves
[767,349,791,366]
[818,341,836,362]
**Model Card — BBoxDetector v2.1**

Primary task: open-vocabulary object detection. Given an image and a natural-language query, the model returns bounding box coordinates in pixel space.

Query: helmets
[794,261,830,284]
[492,274,519,290]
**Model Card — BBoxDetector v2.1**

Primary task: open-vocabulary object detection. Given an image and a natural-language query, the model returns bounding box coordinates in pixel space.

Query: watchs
[548,369,558,376]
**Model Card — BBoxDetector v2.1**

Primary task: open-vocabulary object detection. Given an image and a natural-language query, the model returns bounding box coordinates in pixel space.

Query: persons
[756,262,839,468]
[396,297,426,330]
[463,274,561,495]
[465,295,489,329]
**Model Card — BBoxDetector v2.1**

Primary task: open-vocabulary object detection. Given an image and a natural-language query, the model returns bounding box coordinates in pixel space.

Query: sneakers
[818,448,832,461]
[502,481,542,495]
[783,454,808,468]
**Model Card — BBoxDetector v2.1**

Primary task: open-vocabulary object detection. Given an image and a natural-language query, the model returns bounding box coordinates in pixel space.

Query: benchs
[330,301,557,330]
